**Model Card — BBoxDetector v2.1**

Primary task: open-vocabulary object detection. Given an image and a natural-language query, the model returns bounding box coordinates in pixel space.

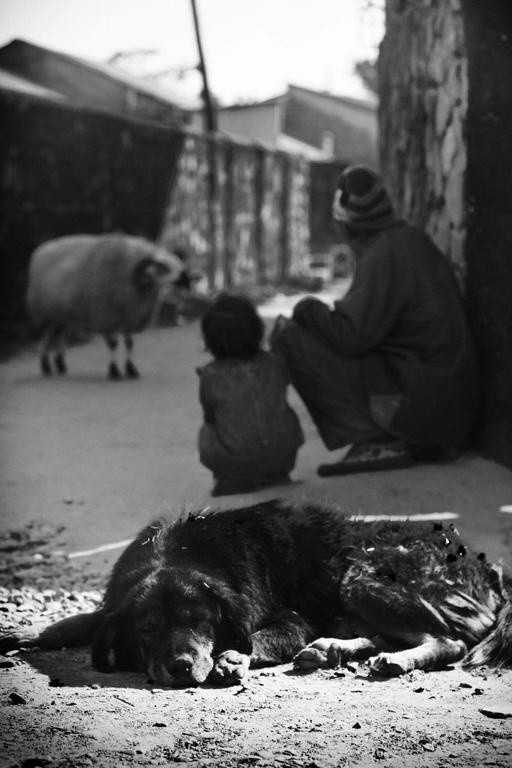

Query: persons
[193,292,301,495]
[266,161,480,473]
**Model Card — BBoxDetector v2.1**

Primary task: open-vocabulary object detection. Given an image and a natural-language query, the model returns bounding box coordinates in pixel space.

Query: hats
[332,164,395,230]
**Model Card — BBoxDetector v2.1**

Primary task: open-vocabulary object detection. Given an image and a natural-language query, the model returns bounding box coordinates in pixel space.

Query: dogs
[89,488,470,688]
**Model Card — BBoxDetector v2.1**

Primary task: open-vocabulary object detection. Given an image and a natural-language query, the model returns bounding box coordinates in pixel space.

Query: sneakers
[318,441,415,476]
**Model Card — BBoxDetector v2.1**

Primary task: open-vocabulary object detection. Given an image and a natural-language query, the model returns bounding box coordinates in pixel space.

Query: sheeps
[28,232,184,380]
